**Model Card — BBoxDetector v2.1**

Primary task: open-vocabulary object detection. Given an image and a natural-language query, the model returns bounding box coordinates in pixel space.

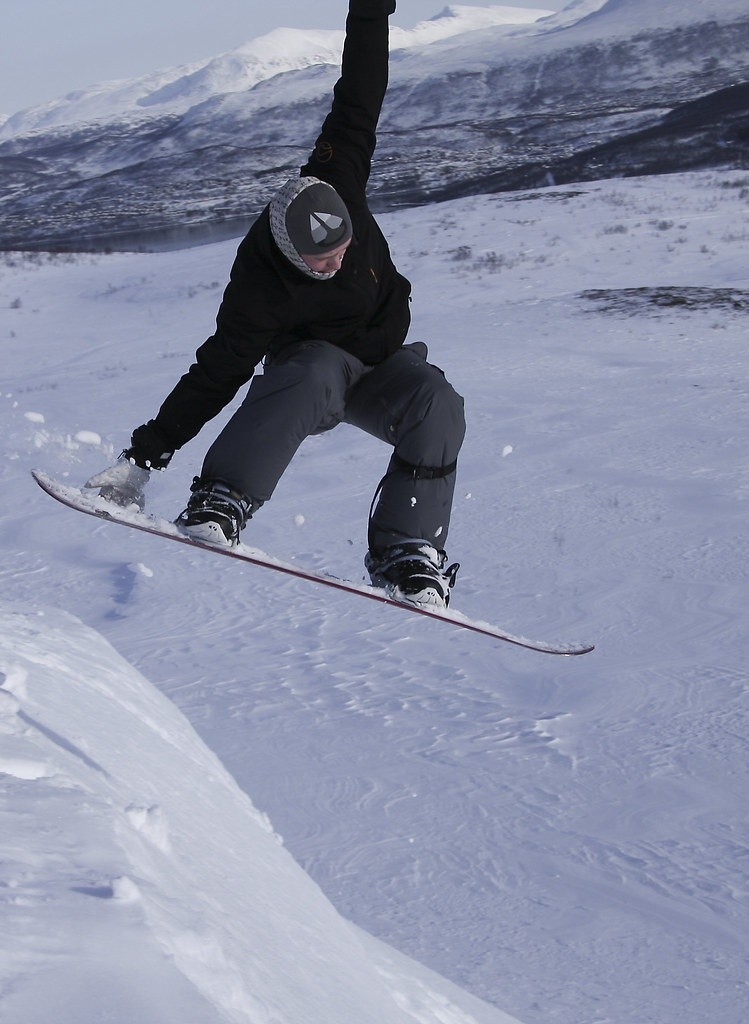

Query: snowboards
[27,467,595,654]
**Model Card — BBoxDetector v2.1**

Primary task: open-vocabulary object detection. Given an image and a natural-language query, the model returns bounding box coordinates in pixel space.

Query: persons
[85,0,465,604]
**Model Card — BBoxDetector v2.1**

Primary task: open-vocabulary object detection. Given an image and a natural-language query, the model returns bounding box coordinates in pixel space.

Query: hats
[269,175,353,255]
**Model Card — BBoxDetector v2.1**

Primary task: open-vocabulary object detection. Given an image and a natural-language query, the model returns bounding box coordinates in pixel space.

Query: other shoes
[364,539,459,608]
[174,475,262,546]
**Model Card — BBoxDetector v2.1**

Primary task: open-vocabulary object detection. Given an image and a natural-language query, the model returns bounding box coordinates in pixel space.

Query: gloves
[85,456,150,511]
